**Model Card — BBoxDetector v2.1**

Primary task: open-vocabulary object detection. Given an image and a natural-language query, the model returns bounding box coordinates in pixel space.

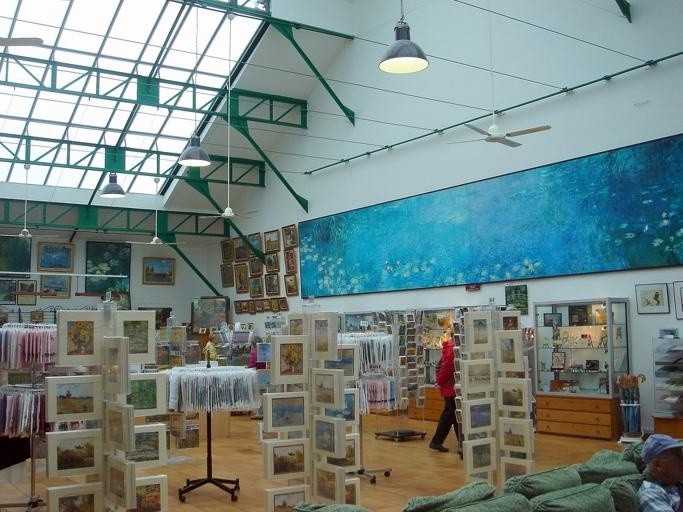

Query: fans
[447,0,551,148]
[0,161,59,238]
[127,153,185,245]
[199,8,257,225]
[197,172,260,219]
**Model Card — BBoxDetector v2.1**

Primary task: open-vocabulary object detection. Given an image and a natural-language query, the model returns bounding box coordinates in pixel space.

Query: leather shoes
[429,443,449,452]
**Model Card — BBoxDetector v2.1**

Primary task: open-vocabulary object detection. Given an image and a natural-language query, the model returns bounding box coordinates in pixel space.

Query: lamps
[176,0,211,166]
[376,0,429,74]
[100,118,126,199]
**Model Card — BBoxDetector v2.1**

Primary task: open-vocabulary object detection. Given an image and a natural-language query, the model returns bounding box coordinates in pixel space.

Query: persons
[637,430,683,512]
[204,336,216,361]
[249,337,266,368]
[429,332,465,451]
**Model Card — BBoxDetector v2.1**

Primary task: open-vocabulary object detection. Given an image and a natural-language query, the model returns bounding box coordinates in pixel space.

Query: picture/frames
[460,359,496,393]
[320,388,359,426]
[502,356,529,378]
[284,250,296,272]
[263,390,309,432]
[269,335,309,385]
[544,312,562,328]
[501,457,534,489]
[191,297,227,333]
[416,325,423,336]
[408,369,417,376]
[118,374,168,416]
[399,325,406,335]
[114,311,155,364]
[402,387,409,398]
[279,297,287,311]
[37,242,74,271]
[264,253,280,272]
[409,382,418,390]
[452,320,459,334]
[399,355,406,365]
[312,368,345,410]
[249,277,264,298]
[418,384,426,397]
[267,483,311,512]
[143,257,175,285]
[454,332,460,346]
[18,280,37,306]
[313,415,346,458]
[400,364,408,377]
[152,310,198,463]
[314,462,346,503]
[271,297,279,312]
[497,377,533,412]
[264,230,280,251]
[297,131,683,300]
[284,274,299,295]
[264,438,309,479]
[106,456,137,509]
[454,396,462,410]
[406,314,415,322]
[417,376,425,385]
[385,324,392,334]
[408,389,418,399]
[235,300,242,313]
[220,239,234,263]
[454,369,461,383]
[459,333,466,345]
[462,438,495,473]
[40,275,71,298]
[289,479,309,485]
[418,356,425,366]
[406,335,416,342]
[398,315,405,324]
[407,341,416,349]
[118,424,167,469]
[505,285,528,315]
[0,235,31,277]
[254,299,263,312]
[234,263,249,294]
[674,280,683,322]
[104,337,130,395]
[264,273,279,295]
[320,433,360,472]
[417,335,423,345]
[460,326,465,336]
[377,321,386,330]
[0,280,16,305]
[257,370,272,390]
[452,344,460,358]
[408,354,416,363]
[256,343,271,362]
[499,416,534,453]
[85,240,131,293]
[281,224,298,247]
[408,327,416,336]
[407,320,415,329]
[400,398,409,410]
[232,236,248,262]
[409,375,418,384]
[635,284,670,314]
[460,344,466,358]
[454,357,460,372]
[45,376,105,423]
[203,333,255,366]
[399,336,407,347]
[400,377,408,388]
[453,384,463,396]
[221,264,233,287]
[311,312,337,362]
[461,317,464,326]
[343,311,389,333]
[408,361,415,370]
[465,312,493,353]
[247,232,261,256]
[417,346,423,356]
[263,299,271,311]
[407,347,417,355]
[318,344,358,382]
[240,300,248,312]
[46,482,105,512]
[283,383,306,390]
[249,257,262,275]
[544,313,561,327]
[46,429,104,478]
[56,310,102,367]
[498,310,522,330]
[397,347,406,356]
[122,475,168,512]
[344,478,361,506]
[106,403,136,453]
[418,367,426,376]
[229,319,280,335]
[416,395,426,409]
[138,307,172,331]
[455,408,462,423]
[276,432,307,438]
[30,310,43,322]
[461,398,495,433]
[286,313,304,334]
[248,299,256,314]
[496,331,524,372]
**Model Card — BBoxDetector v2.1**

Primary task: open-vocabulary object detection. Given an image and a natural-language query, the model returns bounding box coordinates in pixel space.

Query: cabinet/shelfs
[0,320,61,512]
[374,308,427,442]
[533,299,628,441]
[653,338,683,436]
[407,307,464,422]
[158,365,260,504]
